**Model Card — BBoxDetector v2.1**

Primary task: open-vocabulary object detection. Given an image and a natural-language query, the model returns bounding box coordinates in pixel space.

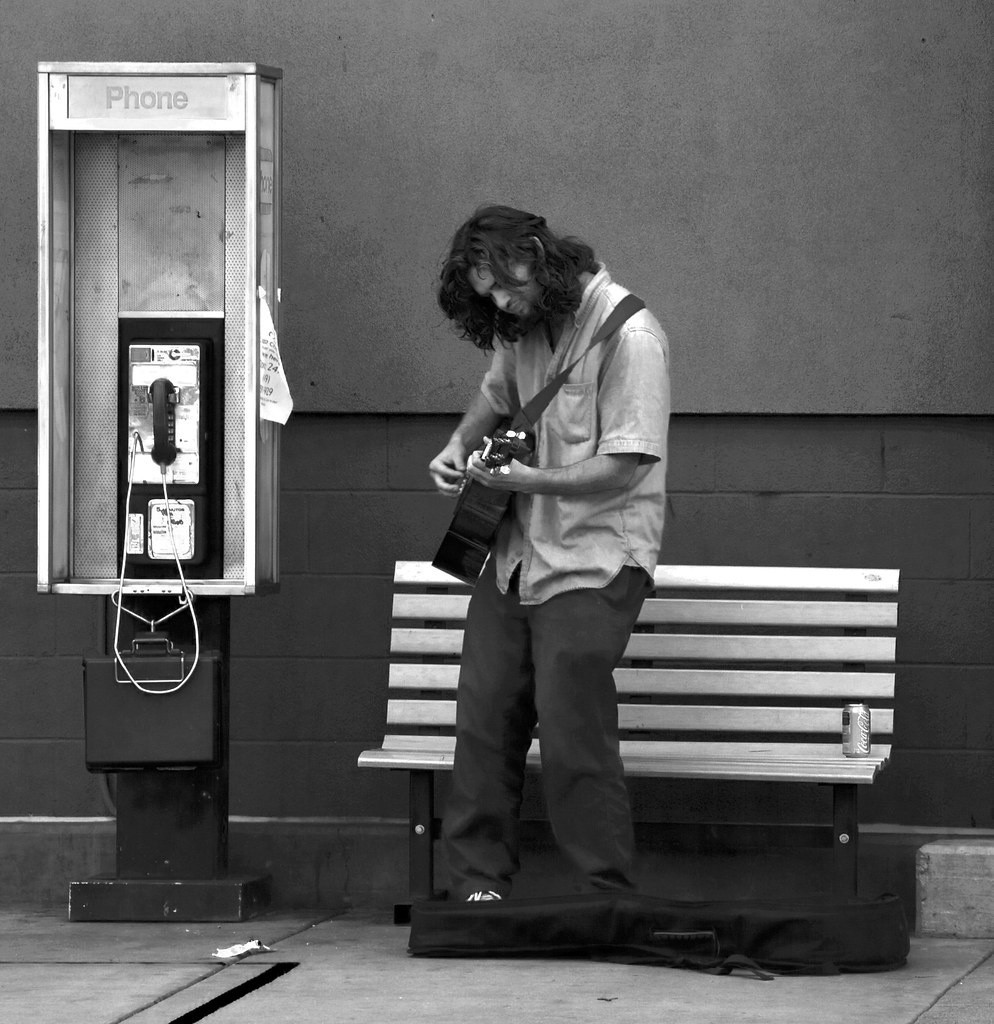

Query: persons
[427,205,671,904]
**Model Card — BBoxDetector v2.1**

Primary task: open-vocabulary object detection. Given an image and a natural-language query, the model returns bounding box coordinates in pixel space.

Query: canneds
[842,703,871,759]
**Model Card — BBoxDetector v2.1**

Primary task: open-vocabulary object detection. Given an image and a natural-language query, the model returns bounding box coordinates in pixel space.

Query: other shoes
[466,889,503,902]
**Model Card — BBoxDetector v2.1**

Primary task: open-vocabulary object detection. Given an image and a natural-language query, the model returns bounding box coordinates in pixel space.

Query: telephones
[114,310,223,580]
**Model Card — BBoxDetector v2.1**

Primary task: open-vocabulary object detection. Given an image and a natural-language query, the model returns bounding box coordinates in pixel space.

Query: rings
[489,469,493,474]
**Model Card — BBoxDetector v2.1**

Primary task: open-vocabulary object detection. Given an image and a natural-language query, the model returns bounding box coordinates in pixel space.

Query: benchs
[357,558,900,924]
